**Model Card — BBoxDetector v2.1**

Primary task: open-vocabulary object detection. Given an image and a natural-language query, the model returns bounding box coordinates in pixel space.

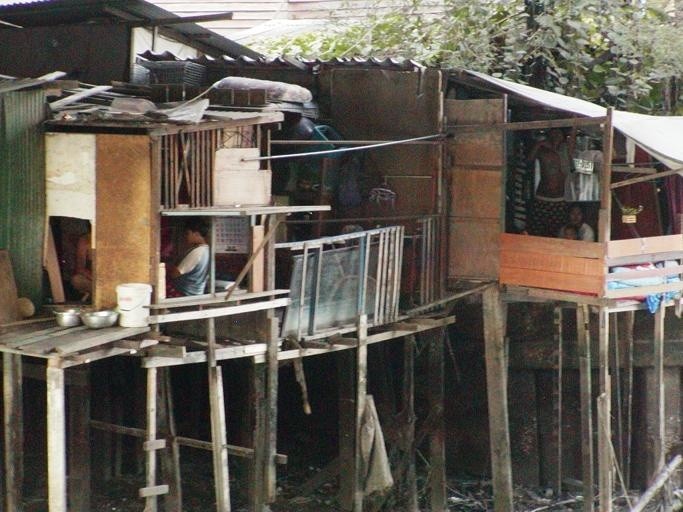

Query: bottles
[158,262,165,301]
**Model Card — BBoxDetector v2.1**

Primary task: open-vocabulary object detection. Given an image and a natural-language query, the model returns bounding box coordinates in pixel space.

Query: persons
[70,223,92,302]
[525,127,596,240]
[166,217,211,297]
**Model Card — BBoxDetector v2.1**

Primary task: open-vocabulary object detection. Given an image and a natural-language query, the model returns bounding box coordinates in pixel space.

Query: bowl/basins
[80,311,118,328]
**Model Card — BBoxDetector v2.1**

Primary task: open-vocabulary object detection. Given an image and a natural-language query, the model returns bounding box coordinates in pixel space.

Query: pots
[52,308,84,327]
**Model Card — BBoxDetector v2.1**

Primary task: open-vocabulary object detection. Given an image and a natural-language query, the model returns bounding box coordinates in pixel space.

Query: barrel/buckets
[116,282,153,327]
